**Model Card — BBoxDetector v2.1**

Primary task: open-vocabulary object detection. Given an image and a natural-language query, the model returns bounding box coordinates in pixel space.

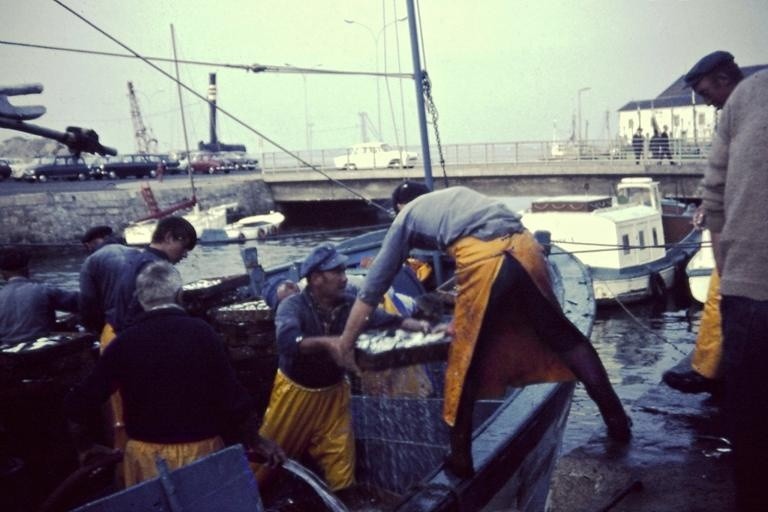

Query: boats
[123,200,286,246]
[1,216,596,512]
[516,179,704,309]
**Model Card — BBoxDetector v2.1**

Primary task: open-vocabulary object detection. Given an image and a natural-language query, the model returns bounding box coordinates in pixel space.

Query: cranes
[127,83,157,152]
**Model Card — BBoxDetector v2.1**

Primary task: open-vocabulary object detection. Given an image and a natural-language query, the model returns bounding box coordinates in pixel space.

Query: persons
[647,129,660,159]
[241,241,434,509]
[74,220,148,491]
[239,245,302,312]
[655,124,677,166]
[56,258,291,497]
[332,176,634,478]
[660,49,747,396]
[631,126,646,165]
[92,212,200,495]
[0,240,87,468]
[700,61,768,511]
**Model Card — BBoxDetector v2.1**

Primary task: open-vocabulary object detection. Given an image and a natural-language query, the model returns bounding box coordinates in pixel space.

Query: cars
[91,152,258,181]
[335,142,417,170]
[0,154,91,182]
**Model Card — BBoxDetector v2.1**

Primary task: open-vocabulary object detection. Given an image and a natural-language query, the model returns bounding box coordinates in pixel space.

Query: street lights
[285,64,326,151]
[577,86,594,156]
[345,16,408,142]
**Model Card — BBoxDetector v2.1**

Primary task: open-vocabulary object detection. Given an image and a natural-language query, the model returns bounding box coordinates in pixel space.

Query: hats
[682,51,735,90]
[298,242,349,278]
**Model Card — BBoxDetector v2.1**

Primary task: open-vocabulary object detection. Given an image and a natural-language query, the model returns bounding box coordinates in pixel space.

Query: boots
[444,398,474,474]
[586,377,632,440]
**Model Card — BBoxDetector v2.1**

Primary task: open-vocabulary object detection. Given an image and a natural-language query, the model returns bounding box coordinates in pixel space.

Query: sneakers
[663,369,717,395]
